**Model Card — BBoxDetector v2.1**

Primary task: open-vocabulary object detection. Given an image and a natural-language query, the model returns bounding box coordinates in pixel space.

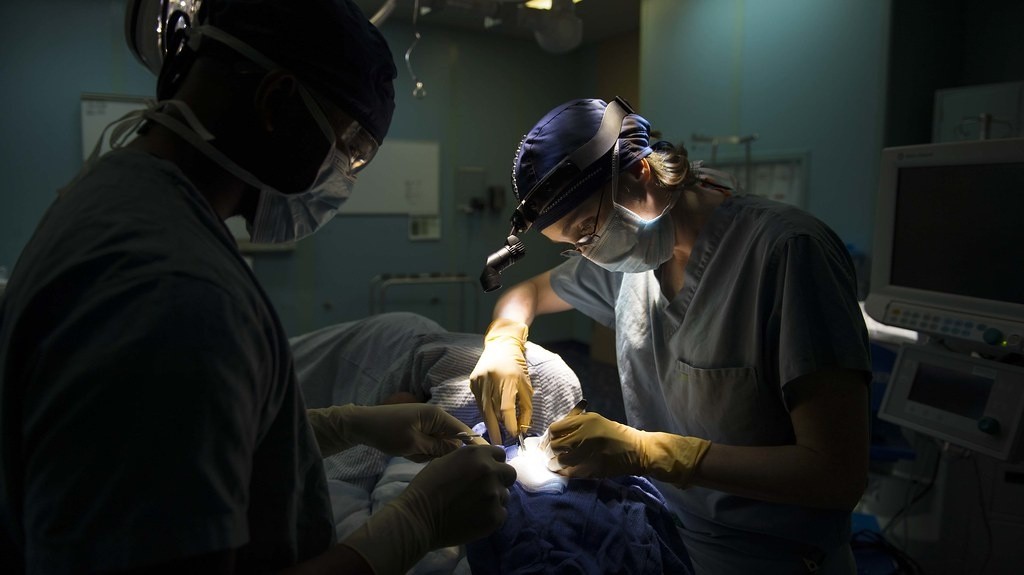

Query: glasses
[301,84,379,176]
[559,186,605,258]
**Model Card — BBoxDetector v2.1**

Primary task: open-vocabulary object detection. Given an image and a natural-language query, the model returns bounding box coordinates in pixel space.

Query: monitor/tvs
[864,138,1024,348]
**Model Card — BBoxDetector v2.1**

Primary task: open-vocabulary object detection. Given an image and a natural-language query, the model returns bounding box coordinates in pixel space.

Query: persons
[468,98,868,575]
[0,0,516,575]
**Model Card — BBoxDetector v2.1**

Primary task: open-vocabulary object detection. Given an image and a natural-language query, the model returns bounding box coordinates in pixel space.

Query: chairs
[869,342,917,460]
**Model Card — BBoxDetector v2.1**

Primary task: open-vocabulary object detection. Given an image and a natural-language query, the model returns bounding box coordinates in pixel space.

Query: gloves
[552,412,712,490]
[304,403,488,463]
[469,318,534,445]
[339,445,517,575]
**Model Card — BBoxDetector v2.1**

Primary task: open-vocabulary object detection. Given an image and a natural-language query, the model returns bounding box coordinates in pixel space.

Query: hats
[510,98,654,233]
[198,0,397,147]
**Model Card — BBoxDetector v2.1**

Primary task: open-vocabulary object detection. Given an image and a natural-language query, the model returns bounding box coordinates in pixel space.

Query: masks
[251,140,356,245]
[581,205,676,273]
